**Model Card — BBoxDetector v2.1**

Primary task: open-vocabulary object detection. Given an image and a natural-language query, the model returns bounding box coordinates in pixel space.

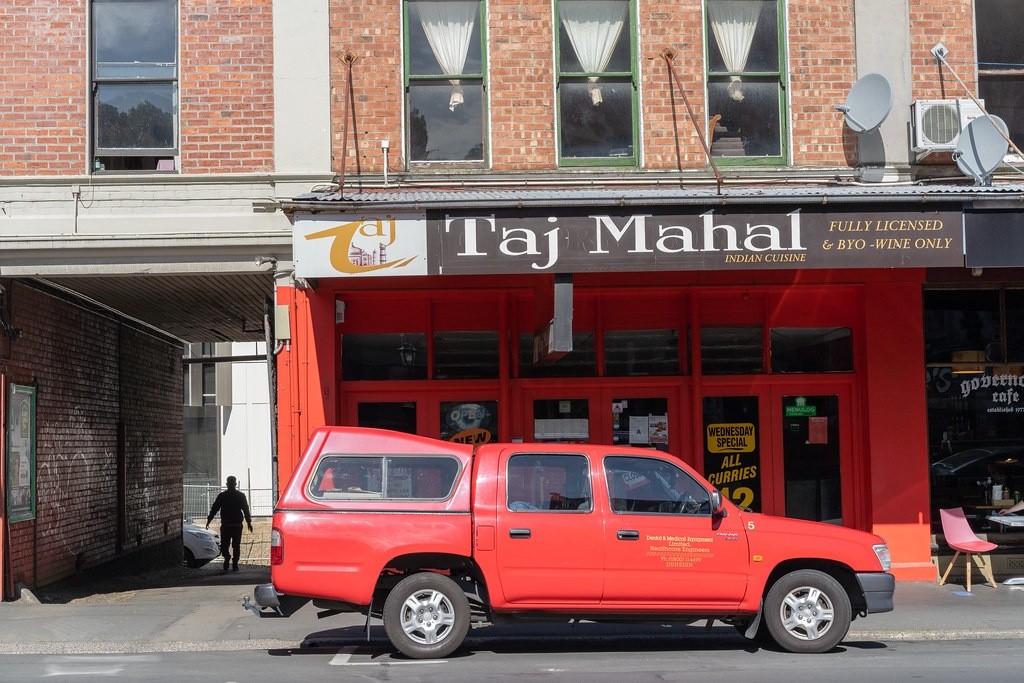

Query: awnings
[263,47,1024,218]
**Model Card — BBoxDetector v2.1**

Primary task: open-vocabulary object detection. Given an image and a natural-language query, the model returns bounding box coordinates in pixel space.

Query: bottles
[1013,491,1020,505]
[1003,487,1009,500]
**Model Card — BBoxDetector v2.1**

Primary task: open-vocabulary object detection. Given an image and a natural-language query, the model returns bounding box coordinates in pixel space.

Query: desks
[987,514,1024,586]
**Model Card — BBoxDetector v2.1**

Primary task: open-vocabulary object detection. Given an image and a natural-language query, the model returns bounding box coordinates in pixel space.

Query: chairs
[939,506,999,593]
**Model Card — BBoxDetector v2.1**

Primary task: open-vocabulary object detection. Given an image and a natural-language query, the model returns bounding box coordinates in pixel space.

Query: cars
[183,520,223,570]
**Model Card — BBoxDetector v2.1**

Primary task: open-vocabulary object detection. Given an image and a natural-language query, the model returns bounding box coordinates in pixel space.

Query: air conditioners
[911,99,983,155]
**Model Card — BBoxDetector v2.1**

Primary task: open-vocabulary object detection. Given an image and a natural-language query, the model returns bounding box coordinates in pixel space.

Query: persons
[1000,495,1024,517]
[205,476,253,570]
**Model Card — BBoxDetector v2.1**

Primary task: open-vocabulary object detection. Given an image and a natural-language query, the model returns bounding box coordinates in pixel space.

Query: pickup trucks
[236,424,897,660]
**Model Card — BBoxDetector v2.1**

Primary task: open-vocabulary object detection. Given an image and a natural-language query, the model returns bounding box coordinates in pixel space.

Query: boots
[223,555,239,572]
